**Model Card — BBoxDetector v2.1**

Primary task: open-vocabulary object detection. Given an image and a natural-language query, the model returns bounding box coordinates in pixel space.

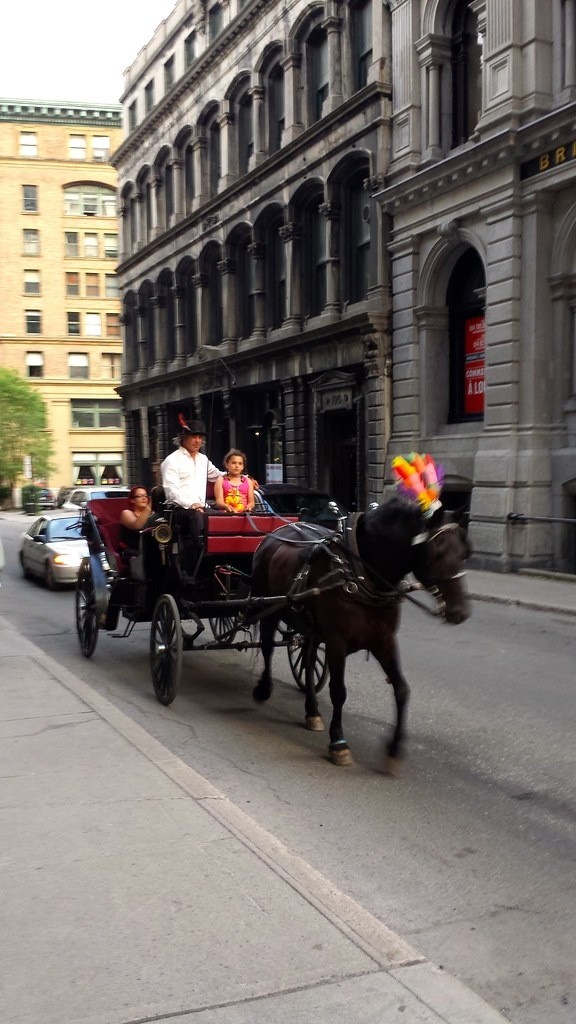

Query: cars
[19,512,115,593]
[23,485,130,517]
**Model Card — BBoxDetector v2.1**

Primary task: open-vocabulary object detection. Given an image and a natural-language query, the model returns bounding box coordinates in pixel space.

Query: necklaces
[237,479,239,493]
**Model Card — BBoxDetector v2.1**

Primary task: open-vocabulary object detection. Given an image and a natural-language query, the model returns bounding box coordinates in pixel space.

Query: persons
[121,486,153,553]
[214,448,255,512]
[159,421,258,572]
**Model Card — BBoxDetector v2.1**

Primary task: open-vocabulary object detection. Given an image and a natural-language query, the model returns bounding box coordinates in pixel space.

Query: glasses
[133,494,148,498]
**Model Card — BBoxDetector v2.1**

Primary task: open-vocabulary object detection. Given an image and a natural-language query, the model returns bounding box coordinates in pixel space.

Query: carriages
[64,450,473,779]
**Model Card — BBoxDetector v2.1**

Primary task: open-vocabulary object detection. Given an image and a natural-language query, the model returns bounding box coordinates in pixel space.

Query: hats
[178,414,207,435]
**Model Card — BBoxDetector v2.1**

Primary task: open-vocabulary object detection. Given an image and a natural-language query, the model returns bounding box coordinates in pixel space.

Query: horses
[251,495,471,778]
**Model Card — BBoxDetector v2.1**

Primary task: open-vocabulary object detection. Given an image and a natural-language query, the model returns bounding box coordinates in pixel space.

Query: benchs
[86,496,154,569]
[205,481,264,513]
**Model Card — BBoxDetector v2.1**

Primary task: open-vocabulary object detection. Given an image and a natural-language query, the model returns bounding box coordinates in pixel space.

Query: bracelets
[145,508,152,511]
